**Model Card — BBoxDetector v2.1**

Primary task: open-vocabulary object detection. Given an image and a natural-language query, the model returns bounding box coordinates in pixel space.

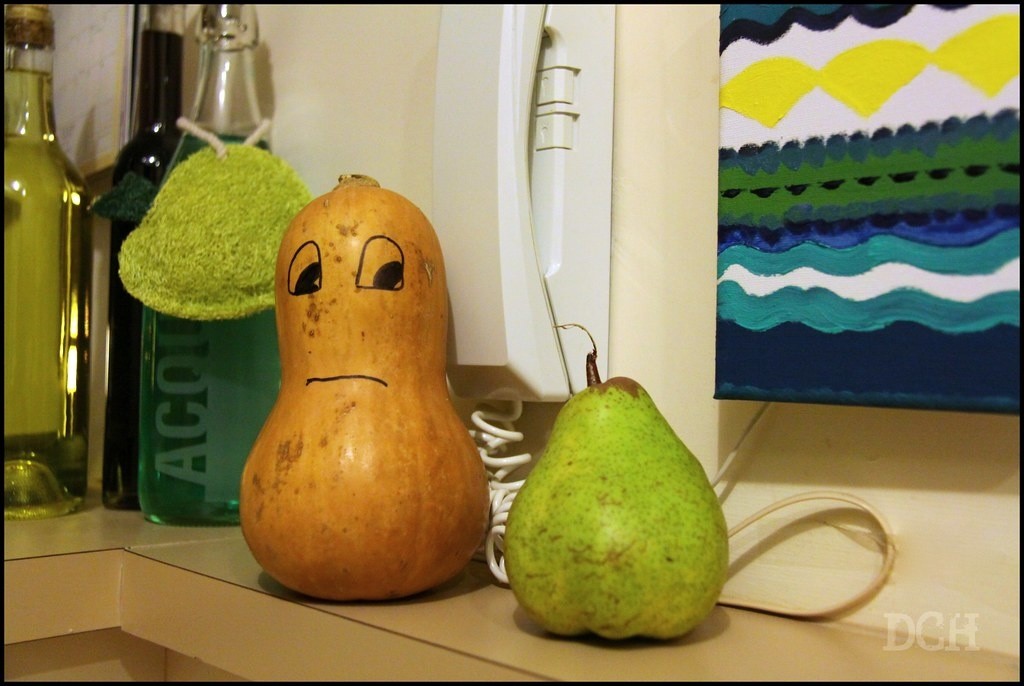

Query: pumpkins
[237,171,491,599]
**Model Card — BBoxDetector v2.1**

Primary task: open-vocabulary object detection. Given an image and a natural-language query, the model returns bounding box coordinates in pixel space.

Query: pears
[497,350,733,641]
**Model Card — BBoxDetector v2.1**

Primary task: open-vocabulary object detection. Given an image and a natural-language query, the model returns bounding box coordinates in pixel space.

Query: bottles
[4,4,99,520]
[89,3,209,510]
[133,4,289,522]
